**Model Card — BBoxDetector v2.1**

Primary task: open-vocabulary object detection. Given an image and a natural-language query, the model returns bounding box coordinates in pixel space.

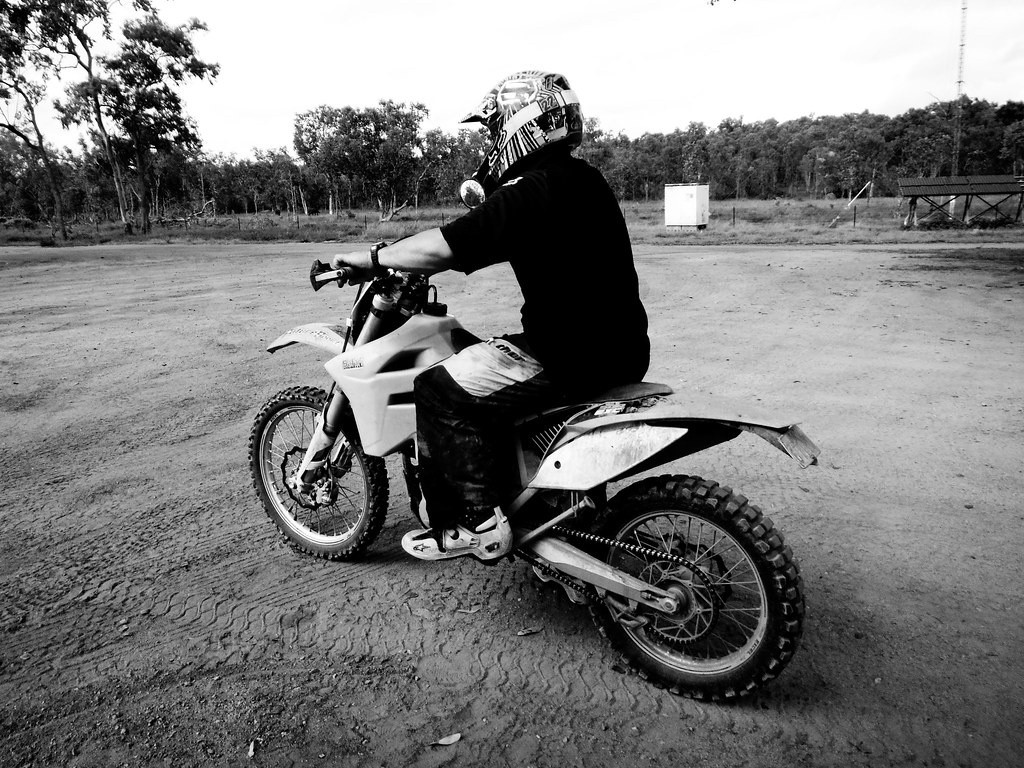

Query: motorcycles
[246,180,824,702]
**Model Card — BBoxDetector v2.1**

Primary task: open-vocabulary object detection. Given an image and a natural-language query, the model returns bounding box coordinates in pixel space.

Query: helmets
[461,68,584,188]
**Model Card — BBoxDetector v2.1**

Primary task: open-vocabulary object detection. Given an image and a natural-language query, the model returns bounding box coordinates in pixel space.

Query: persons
[465,187,481,208]
[333,71,650,560]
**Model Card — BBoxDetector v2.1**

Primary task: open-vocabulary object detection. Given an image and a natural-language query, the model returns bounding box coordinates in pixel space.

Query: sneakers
[400,505,516,566]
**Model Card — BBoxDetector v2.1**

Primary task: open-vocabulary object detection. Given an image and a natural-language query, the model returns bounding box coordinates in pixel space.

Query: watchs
[370,242,388,268]
